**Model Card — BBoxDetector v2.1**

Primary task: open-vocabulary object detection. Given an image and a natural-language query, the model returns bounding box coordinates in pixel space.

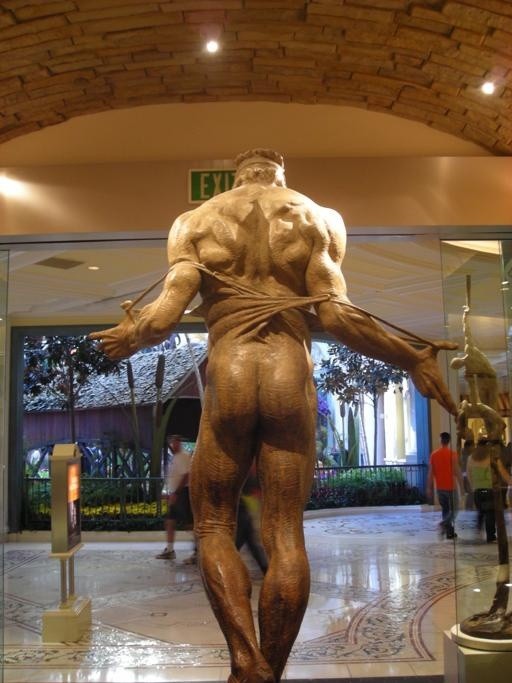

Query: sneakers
[155,546,177,559]
[182,550,198,565]
[440,521,457,538]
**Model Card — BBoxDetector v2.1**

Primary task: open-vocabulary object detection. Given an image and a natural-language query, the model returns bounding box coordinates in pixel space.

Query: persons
[234,468,270,577]
[154,433,198,564]
[86,148,465,681]
[467,435,511,541]
[425,431,462,539]
